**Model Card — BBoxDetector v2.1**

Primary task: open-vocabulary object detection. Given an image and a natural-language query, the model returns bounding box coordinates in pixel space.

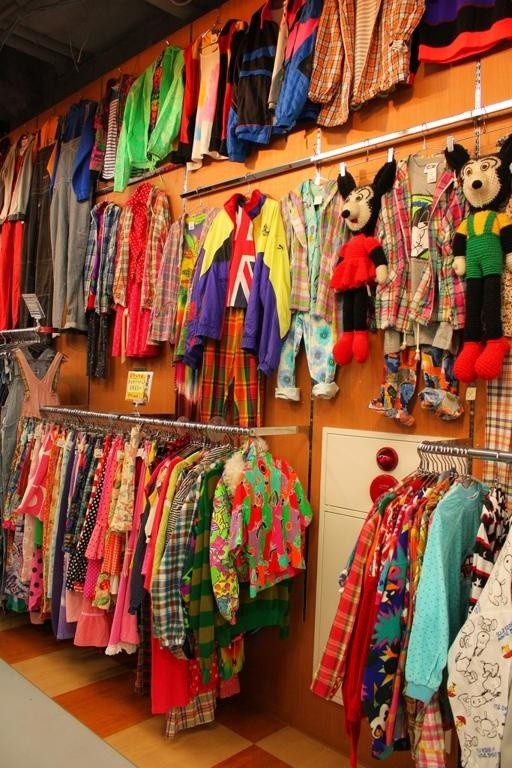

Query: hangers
[1,325,74,364]
[303,144,333,192]
[197,9,225,50]
[413,441,505,490]
[188,189,214,220]
[410,122,442,163]
[97,187,115,213]
[33,408,258,453]
[237,175,260,208]
[136,172,157,193]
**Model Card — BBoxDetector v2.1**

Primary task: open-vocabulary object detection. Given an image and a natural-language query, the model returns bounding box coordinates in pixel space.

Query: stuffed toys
[328,158,397,366]
[443,132,510,383]
